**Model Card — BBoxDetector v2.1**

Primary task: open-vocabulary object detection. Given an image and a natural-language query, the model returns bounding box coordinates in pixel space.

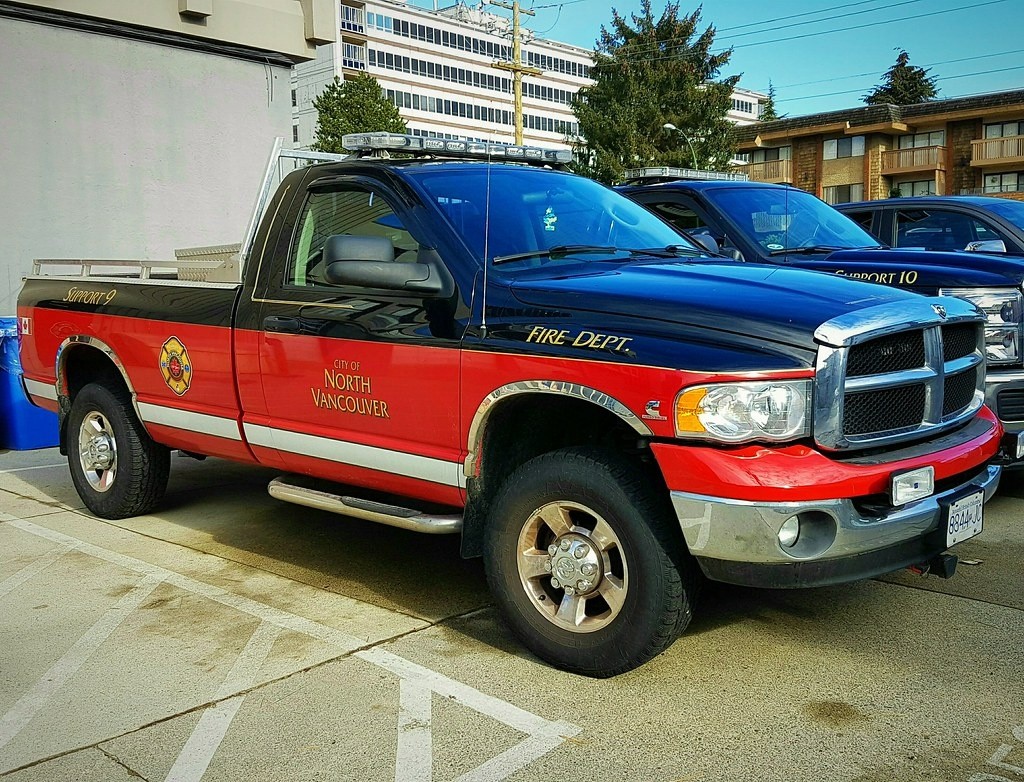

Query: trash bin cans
[0,315,61,452]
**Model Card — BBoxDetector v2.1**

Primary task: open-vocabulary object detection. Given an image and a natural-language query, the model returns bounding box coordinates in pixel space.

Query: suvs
[372,179,1024,471]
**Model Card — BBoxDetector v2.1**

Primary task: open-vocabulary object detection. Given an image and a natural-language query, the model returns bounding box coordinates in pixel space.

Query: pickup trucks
[12,133,1008,676]
